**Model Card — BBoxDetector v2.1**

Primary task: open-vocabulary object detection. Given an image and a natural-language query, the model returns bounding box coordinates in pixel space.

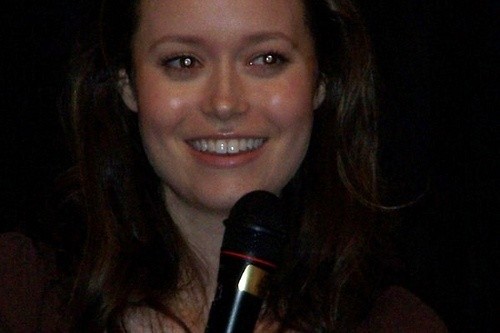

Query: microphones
[203,191,288,333]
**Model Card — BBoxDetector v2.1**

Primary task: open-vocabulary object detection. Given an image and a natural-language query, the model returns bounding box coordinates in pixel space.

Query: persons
[51,0,445,333]
